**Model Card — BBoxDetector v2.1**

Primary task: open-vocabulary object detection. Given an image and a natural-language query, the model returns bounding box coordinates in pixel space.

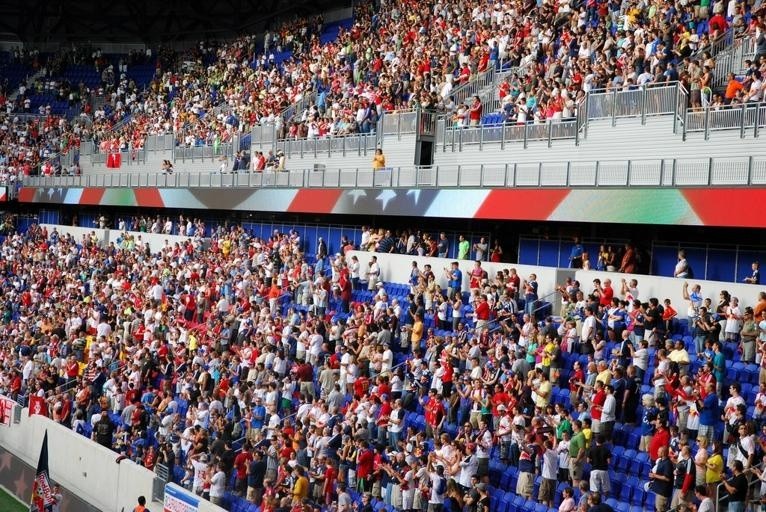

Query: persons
[1,202,766,512]
[1,1,765,186]
